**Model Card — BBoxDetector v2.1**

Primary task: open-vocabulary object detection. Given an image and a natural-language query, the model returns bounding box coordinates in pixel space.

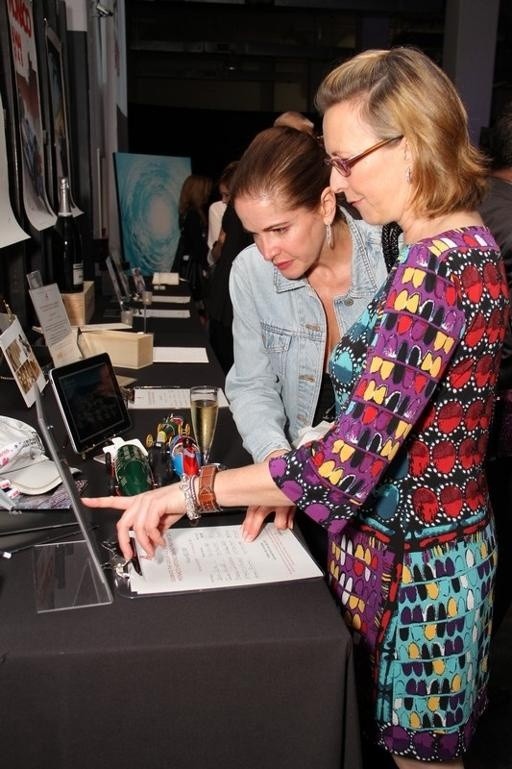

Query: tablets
[49,353,135,455]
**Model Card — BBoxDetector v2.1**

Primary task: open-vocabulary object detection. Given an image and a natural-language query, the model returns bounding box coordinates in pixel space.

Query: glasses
[323,134,404,177]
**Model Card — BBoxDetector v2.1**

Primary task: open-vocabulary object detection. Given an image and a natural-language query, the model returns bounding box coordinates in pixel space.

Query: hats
[0,415,82,496]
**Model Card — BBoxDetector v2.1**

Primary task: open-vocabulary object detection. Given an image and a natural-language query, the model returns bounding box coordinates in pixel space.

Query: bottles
[54,177,85,294]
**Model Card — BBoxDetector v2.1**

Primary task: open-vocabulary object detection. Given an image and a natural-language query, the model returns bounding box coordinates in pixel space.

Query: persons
[472,114,512,370]
[82,47,477,769]
[224,130,402,542]
[171,110,316,368]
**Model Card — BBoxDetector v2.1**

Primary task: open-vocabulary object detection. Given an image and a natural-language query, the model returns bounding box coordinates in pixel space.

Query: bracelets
[178,472,203,521]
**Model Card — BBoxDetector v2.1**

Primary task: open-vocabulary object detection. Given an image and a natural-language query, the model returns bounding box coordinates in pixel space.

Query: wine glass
[190,385,219,466]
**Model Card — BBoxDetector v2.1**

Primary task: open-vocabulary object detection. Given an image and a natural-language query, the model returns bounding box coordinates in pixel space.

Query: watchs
[198,463,227,512]
